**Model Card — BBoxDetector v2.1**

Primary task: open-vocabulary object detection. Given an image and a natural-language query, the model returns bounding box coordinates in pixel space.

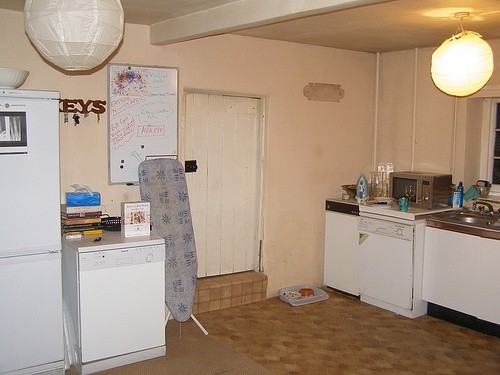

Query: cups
[370,171,385,197]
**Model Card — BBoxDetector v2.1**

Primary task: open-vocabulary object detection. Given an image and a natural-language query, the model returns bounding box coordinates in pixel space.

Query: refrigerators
[0,91,67,375]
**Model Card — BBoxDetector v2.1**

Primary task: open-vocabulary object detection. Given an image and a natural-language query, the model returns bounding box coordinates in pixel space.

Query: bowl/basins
[342,185,356,198]
[299,288,315,297]
[0,67,30,90]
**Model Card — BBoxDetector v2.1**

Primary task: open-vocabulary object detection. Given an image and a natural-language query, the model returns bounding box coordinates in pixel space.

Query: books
[61,204,107,235]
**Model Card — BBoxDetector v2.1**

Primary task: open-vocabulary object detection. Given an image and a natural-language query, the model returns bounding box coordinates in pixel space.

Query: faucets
[472,200,500,217]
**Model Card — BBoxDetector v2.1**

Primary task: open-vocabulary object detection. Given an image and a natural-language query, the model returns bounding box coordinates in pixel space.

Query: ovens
[357,215,427,320]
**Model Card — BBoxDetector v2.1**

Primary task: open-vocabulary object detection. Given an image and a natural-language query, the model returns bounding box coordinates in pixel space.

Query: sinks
[444,208,500,230]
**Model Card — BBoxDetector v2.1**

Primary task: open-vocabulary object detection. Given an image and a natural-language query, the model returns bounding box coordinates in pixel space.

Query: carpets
[65,320,277,375]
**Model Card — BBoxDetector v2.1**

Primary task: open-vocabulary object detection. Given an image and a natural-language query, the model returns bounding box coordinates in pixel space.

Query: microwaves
[389,171,453,210]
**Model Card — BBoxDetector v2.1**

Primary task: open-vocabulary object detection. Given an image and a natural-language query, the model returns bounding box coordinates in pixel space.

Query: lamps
[430,12,494,97]
[23,0,123,72]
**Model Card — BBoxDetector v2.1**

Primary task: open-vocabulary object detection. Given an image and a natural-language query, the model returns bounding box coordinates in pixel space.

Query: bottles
[450,183,456,207]
